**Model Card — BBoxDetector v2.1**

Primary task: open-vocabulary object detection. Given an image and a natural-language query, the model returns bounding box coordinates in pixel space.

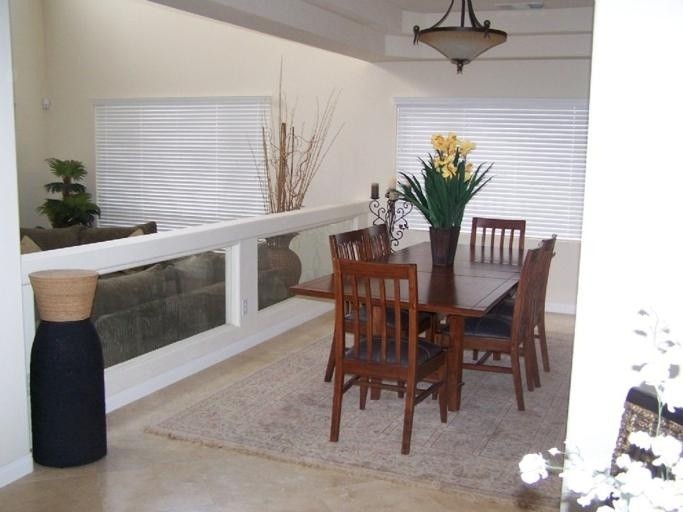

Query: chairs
[328,224,446,454]
[434,217,558,410]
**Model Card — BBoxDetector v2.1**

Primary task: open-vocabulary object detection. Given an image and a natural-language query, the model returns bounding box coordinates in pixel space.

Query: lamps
[413,0,509,74]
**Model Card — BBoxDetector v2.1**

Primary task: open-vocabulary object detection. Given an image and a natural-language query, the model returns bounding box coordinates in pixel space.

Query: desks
[286,241,540,413]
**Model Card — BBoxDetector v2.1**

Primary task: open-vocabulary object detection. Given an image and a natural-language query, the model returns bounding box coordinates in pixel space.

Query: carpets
[140,323,572,506]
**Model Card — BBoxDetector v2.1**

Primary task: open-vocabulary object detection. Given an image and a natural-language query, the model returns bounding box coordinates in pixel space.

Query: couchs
[21,220,289,370]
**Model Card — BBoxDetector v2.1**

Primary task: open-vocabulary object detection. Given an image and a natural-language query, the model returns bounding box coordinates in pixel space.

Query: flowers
[395,131,493,230]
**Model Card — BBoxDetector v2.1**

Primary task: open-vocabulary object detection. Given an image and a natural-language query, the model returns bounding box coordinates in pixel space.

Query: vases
[428,226,459,268]
[266,245,303,288]
